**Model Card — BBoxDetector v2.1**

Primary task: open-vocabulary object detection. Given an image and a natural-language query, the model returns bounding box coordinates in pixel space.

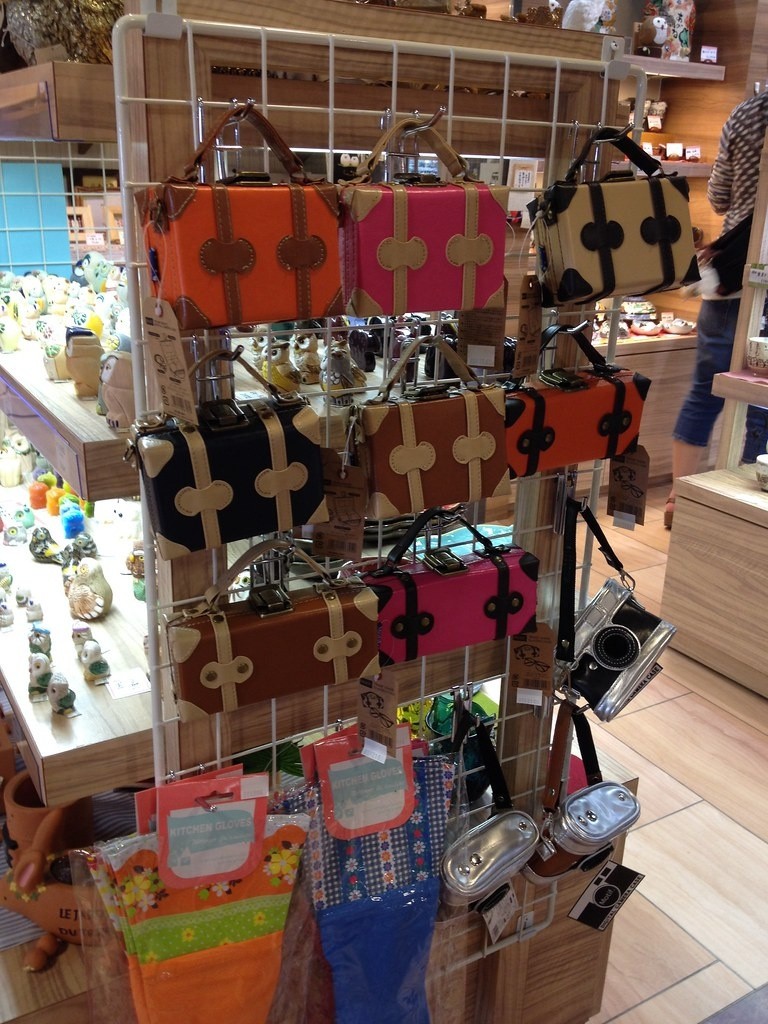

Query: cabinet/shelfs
[611,53,726,177]
[589,335,720,488]
[656,462,768,702]
[0,62,640,1023]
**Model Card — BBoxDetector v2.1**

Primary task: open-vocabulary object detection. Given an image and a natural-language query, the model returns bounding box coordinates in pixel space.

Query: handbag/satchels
[356,335,512,520]
[363,509,540,668]
[681,213,753,297]
[526,127,702,309]
[134,105,344,330]
[500,324,652,480]
[338,117,510,318]
[162,539,381,724]
[123,350,330,561]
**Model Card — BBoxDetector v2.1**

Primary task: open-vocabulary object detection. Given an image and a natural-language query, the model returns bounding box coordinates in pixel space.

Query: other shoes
[664,498,675,525]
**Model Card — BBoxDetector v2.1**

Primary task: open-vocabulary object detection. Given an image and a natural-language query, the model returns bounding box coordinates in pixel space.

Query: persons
[664,88,768,530]
